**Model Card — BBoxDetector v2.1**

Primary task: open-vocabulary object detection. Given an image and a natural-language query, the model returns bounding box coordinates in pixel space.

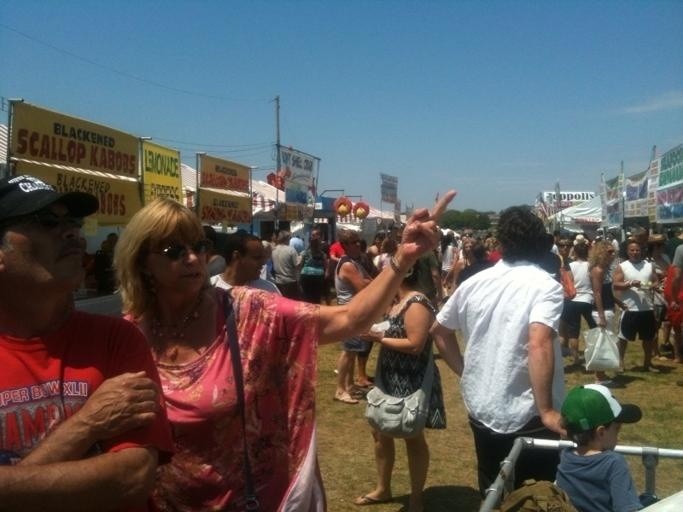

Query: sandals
[335,377,372,403]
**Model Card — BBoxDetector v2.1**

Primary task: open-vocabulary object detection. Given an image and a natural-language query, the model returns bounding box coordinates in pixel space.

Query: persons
[0,174,171,511]
[352,253,448,512]
[77,226,681,407]
[107,188,461,512]
[553,383,660,511]
[427,204,568,512]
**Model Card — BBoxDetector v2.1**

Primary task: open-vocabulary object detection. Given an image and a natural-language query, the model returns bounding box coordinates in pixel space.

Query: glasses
[143,237,214,258]
[15,209,87,231]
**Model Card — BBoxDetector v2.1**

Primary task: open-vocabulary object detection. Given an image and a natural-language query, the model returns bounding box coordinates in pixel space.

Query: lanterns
[332,196,352,219]
[354,201,369,220]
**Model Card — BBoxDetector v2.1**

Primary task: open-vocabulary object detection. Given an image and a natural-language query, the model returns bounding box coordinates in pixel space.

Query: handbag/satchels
[502,477,581,512]
[365,385,428,439]
[300,265,324,278]
[581,326,621,372]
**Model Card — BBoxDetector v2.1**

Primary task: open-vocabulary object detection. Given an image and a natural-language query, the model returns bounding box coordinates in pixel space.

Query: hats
[572,234,590,246]
[561,383,642,433]
[0,175,100,221]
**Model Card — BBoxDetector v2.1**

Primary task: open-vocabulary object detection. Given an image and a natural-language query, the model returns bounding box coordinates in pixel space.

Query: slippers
[351,493,389,505]
